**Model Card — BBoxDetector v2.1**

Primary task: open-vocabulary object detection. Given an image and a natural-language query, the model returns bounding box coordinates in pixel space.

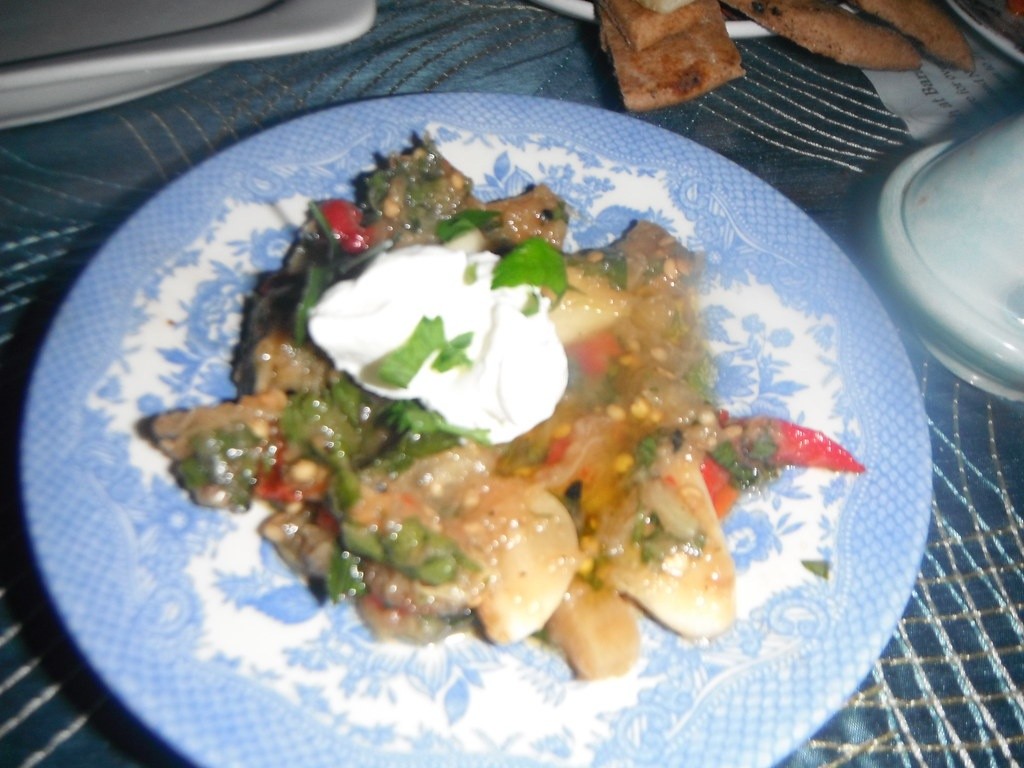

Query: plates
[19,96,931,768]
[530,0,889,41]
[0,0,378,133]
[943,0,1024,72]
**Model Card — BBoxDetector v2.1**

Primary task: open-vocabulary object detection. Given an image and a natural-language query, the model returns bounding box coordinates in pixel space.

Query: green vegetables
[173,200,780,596]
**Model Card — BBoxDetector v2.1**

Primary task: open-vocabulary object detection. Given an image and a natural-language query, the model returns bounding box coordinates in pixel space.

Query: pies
[594,0,977,108]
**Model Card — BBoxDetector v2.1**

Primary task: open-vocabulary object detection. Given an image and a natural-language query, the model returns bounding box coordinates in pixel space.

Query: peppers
[702,417,865,514]
